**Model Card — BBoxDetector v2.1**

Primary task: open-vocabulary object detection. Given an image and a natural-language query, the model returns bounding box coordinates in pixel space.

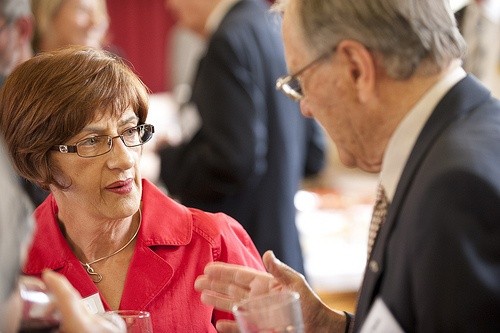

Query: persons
[0,140,128,333]
[193,0,500,333]
[0,0,110,83]
[0,48,272,333]
[164,0,327,287]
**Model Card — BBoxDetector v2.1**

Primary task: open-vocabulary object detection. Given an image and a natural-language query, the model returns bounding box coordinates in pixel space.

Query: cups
[232,291,305,333]
[104,310,154,333]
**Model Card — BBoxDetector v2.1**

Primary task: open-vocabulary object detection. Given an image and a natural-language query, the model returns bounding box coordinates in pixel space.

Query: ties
[366,183,390,258]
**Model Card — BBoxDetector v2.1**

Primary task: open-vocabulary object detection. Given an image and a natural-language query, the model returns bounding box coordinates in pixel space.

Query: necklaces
[78,207,143,283]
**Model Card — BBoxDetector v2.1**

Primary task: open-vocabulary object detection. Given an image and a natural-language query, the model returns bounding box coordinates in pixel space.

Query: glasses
[274,48,337,101]
[51,123,155,158]
